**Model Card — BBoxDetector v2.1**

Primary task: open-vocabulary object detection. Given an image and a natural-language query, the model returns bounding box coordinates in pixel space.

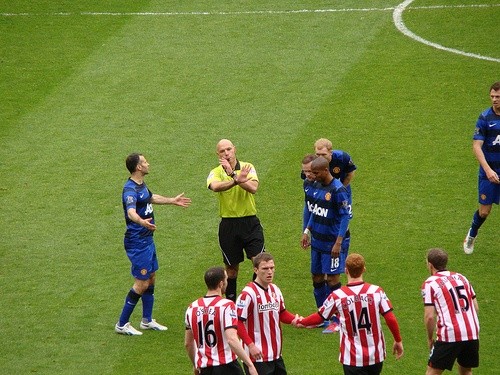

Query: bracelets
[303,228,312,239]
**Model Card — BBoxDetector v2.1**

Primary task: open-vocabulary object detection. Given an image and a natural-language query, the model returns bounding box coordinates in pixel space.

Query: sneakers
[321,321,341,334]
[306,319,330,329]
[463,227,477,255]
[139,318,169,331]
[114,321,143,336]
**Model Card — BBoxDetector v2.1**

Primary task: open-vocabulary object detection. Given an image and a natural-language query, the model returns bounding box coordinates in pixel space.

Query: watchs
[231,171,237,177]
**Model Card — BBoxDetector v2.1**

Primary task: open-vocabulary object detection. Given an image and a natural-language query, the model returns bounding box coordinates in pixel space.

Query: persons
[235,254,318,375]
[463,82,500,255]
[291,253,404,375]
[301,157,350,334]
[301,139,357,320]
[207,139,265,303]
[185,266,259,375]
[114,153,191,336]
[422,249,480,375]
